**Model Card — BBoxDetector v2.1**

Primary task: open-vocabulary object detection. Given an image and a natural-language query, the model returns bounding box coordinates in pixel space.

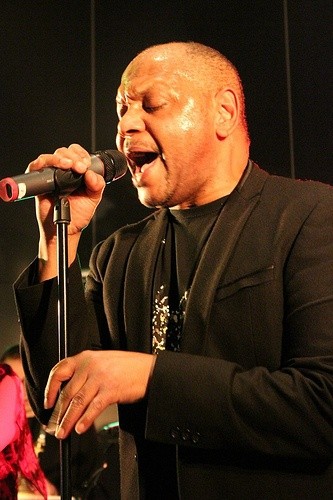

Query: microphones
[0,149,127,202]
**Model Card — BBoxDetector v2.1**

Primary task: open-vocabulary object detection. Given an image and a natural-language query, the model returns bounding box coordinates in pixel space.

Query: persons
[0,343,26,500]
[14,40,333,500]
